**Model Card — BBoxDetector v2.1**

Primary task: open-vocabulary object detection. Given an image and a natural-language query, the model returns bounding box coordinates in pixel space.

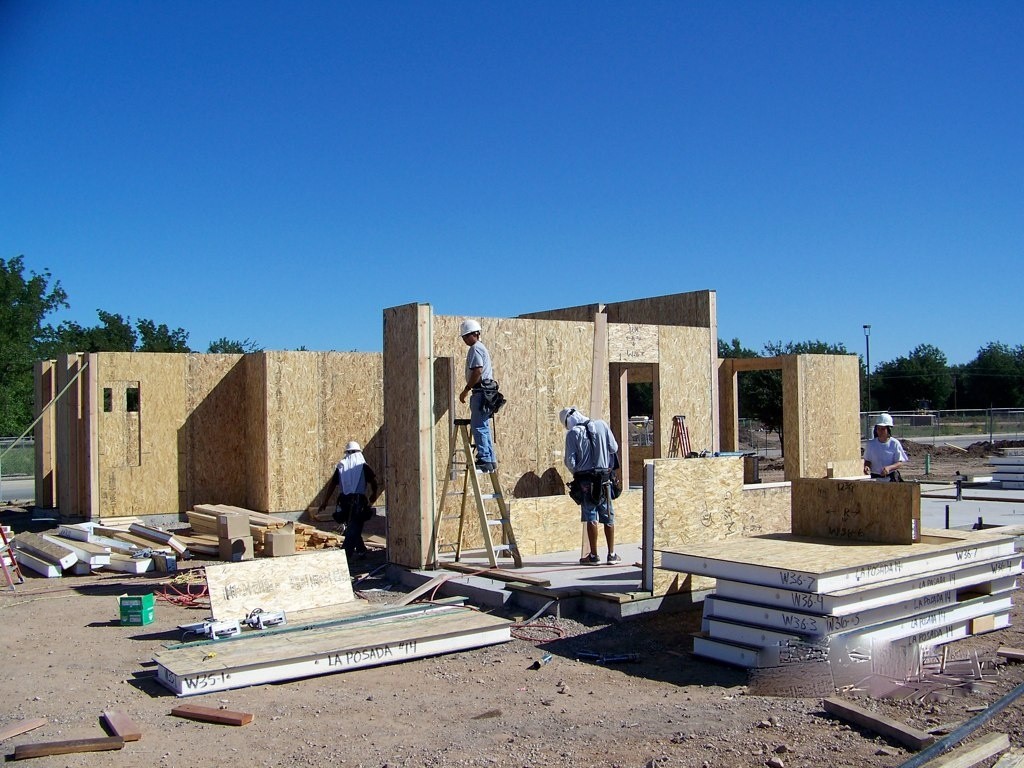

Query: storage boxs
[116,593,157,627]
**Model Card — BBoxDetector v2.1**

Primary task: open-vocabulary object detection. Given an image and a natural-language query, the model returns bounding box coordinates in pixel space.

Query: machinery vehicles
[909,399,936,427]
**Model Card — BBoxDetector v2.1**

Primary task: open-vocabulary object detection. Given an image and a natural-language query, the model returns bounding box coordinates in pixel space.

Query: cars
[631,416,653,434]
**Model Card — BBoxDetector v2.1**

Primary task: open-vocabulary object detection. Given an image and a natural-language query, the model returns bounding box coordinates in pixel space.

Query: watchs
[465,386,471,390]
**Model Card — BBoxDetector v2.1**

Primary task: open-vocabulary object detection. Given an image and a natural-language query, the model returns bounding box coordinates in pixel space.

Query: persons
[559,405,624,566]
[317,440,378,562]
[863,413,908,482]
[457,319,505,473]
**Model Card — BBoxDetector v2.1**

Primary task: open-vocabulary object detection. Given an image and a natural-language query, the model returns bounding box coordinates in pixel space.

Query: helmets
[460,320,481,336]
[875,413,893,427]
[559,407,571,428]
[343,441,363,454]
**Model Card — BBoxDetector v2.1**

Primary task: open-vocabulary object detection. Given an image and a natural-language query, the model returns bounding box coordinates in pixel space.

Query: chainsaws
[193,619,242,641]
[242,608,288,630]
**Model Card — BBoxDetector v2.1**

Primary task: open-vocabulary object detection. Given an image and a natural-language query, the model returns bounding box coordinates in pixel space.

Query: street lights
[864,324,872,412]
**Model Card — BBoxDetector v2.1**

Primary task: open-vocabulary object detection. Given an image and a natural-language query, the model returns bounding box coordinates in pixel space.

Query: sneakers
[607,554,622,564]
[579,554,600,566]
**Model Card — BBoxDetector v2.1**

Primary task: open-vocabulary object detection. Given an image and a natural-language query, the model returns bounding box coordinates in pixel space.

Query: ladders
[0,527,26,592]
[435,418,523,570]
[673,415,692,459]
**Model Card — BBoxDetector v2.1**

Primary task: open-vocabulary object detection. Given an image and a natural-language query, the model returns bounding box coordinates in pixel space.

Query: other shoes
[466,460,497,470]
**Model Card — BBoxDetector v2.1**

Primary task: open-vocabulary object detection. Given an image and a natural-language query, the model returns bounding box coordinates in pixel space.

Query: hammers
[602,481,612,519]
